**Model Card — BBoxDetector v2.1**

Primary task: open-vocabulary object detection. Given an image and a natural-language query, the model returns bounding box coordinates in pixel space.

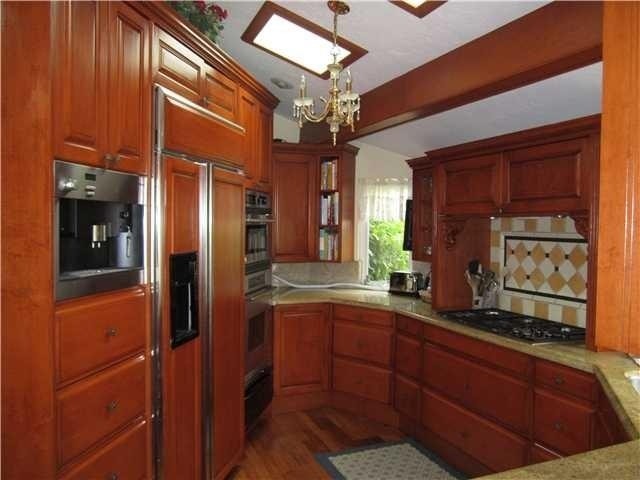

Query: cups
[473,292,496,308]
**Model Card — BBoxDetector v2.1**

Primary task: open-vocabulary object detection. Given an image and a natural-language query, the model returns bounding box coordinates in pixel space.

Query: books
[320,159,339,261]
[248,228,267,258]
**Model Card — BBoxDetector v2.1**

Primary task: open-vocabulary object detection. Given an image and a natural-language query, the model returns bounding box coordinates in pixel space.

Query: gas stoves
[442,305,587,346]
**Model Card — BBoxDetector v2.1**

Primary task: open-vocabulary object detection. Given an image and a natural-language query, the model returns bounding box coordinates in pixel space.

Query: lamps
[290,1,361,149]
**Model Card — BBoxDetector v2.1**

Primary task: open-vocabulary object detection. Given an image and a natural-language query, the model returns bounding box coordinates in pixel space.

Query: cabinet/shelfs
[403,111,600,350]
[274,302,637,480]
[274,144,360,264]
[0,0,273,480]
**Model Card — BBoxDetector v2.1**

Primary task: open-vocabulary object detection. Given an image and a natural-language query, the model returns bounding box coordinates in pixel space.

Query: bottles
[115,225,134,269]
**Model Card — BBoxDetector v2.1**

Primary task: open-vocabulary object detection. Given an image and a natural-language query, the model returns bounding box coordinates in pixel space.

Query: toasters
[389,270,424,294]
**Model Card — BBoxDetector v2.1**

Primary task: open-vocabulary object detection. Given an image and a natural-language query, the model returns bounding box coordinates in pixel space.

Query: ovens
[244,188,274,426]
